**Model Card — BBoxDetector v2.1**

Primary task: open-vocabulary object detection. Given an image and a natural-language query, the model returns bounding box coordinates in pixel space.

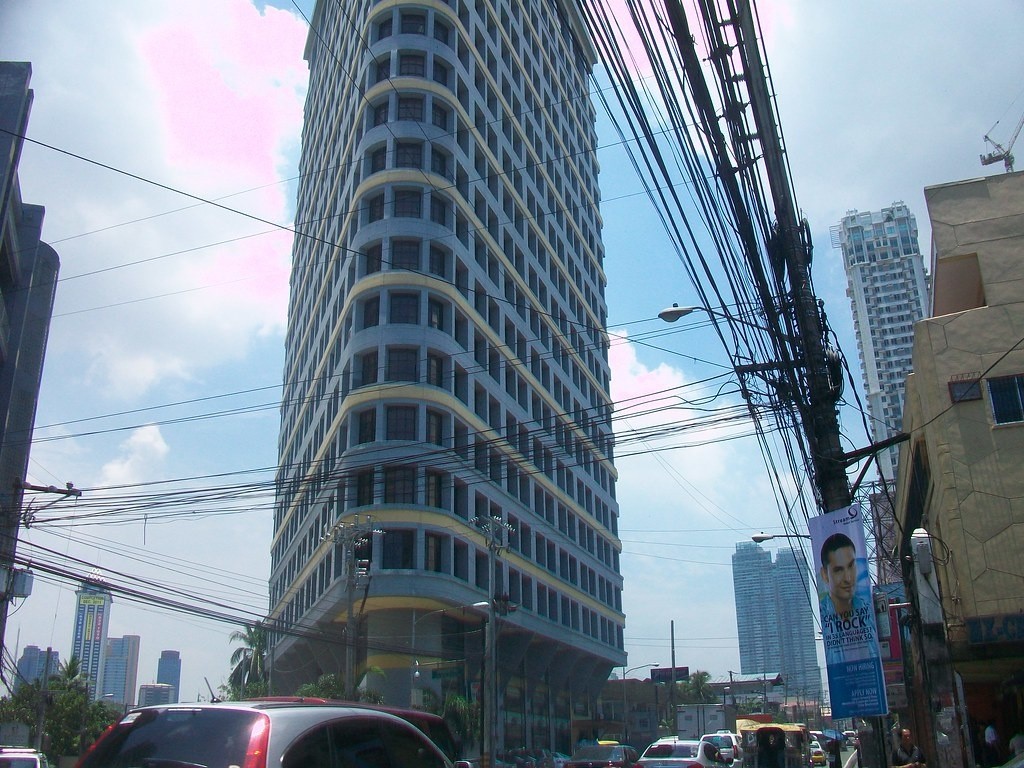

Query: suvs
[700,732,743,768]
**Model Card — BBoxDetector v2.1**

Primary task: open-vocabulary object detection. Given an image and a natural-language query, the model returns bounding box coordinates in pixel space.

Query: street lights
[723,687,730,705]
[409,601,489,707]
[657,306,893,768]
[623,662,660,743]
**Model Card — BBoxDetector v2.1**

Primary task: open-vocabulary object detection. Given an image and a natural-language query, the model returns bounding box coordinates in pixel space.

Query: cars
[809,728,856,768]
[469,748,568,768]
[0,746,50,768]
[71,702,479,768]
[633,736,735,768]
[561,743,640,768]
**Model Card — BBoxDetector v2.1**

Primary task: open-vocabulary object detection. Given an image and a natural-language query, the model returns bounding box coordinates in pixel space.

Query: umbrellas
[822,728,843,740]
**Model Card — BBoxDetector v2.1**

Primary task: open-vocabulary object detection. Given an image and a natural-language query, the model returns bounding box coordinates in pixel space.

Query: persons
[818,534,880,666]
[854,740,862,768]
[978,719,1024,767]
[828,738,843,768]
[889,729,928,768]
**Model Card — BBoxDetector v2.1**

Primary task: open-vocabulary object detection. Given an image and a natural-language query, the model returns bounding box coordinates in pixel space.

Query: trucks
[676,703,738,740]
[741,723,805,768]
